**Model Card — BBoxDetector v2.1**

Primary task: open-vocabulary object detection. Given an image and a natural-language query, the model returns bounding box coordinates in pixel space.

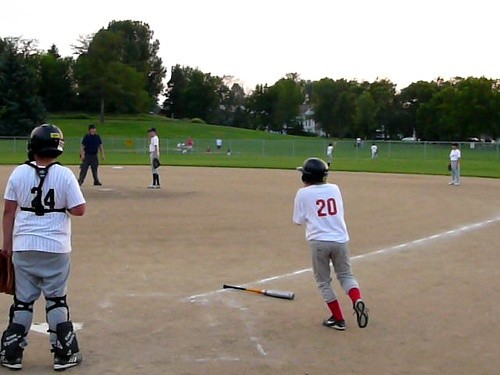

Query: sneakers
[454,183,460,185]
[147,184,156,189]
[53,353,82,369]
[323,315,346,329]
[0,354,22,368]
[94,181,102,185]
[353,299,368,328]
[154,185,160,188]
[448,181,454,185]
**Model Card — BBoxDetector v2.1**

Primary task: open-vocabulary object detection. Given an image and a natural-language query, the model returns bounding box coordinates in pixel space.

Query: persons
[357,136,361,150]
[184,135,233,156]
[293,158,369,331]
[78,124,105,186]
[146,127,161,189]
[326,143,334,166]
[448,143,461,186]
[0,124,86,371]
[370,143,378,159]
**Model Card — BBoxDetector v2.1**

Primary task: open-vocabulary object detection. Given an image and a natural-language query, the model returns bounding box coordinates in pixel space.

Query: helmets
[27,124,64,161]
[297,158,328,174]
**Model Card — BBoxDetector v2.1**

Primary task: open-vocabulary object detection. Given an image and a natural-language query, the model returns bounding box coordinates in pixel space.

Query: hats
[451,143,459,146]
[147,128,156,132]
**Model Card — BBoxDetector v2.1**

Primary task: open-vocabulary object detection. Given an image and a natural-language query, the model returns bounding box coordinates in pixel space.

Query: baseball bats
[223,284,295,299]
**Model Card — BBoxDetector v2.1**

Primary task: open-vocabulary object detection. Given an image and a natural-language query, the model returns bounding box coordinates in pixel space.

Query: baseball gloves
[0,251,14,294]
[448,164,450,170]
[153,158,160,168]
